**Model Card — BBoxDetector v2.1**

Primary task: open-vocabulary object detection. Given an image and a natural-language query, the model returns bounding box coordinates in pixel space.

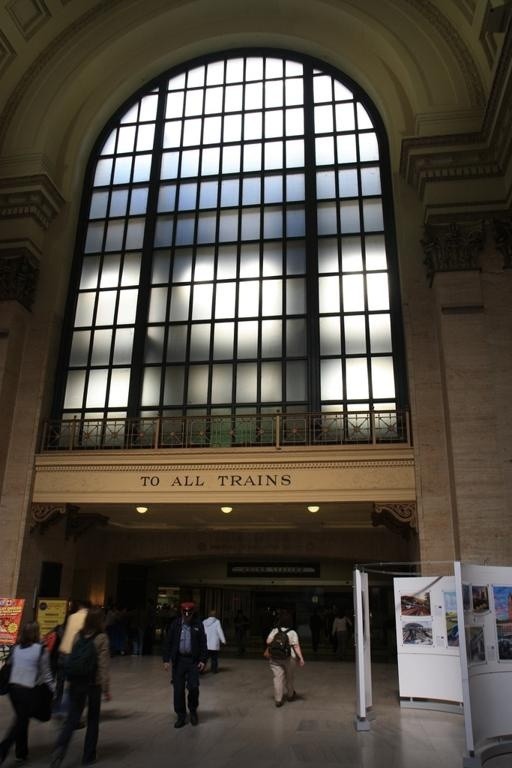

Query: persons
[162,603,207,727]
[0,600,113,768]
[90,603,354,658]
[266,612,305,707]
[201,609,227,676]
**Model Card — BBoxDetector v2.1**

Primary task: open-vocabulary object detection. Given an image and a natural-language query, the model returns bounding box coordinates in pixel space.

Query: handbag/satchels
[0,664,11,696]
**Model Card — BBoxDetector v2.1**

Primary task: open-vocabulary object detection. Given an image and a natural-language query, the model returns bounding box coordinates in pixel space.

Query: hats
[181,603,195,609]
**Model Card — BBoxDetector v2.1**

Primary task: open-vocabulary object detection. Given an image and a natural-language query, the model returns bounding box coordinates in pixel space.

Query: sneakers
[190,713,198,726]
[287,691,296,701]
[276,702,283,707]
[174,717,185,728]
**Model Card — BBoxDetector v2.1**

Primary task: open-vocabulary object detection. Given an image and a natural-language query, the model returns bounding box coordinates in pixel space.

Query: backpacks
[269,626,293,660]
[73,630,101,683]
